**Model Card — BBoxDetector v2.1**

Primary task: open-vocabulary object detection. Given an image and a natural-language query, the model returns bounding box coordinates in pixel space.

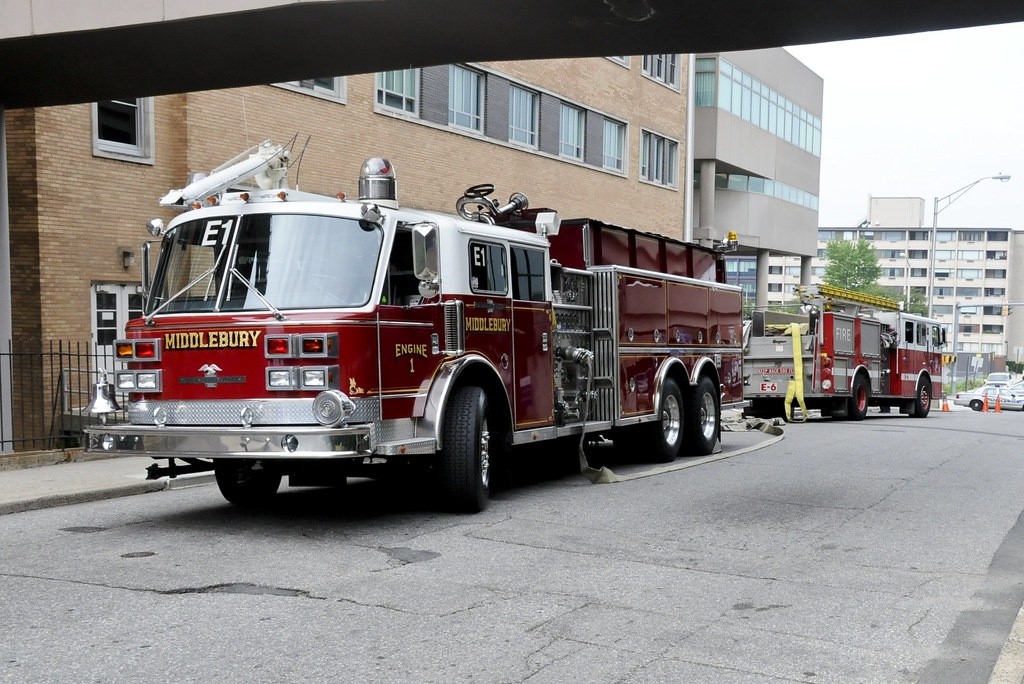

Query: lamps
[123,251,135,267]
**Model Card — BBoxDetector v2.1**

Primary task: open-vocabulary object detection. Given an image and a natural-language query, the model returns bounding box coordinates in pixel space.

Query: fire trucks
[82,132,753,516]
[744,282,946,422]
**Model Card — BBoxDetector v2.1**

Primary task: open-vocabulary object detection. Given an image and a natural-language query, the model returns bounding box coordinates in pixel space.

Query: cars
[953,371,1024,411]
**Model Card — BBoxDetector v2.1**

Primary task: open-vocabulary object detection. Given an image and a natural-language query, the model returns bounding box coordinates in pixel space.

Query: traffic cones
[942,396,952,413]
[980,394,991,413]
[992,394,1004,413]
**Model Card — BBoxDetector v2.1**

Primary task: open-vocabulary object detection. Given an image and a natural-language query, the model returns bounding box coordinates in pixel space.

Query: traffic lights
[1002,299,1013,316]
[941,353,956,367]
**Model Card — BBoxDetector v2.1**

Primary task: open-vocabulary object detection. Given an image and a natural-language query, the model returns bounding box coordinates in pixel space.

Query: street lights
[899,251,910,312]
[927,174,1013,319]
